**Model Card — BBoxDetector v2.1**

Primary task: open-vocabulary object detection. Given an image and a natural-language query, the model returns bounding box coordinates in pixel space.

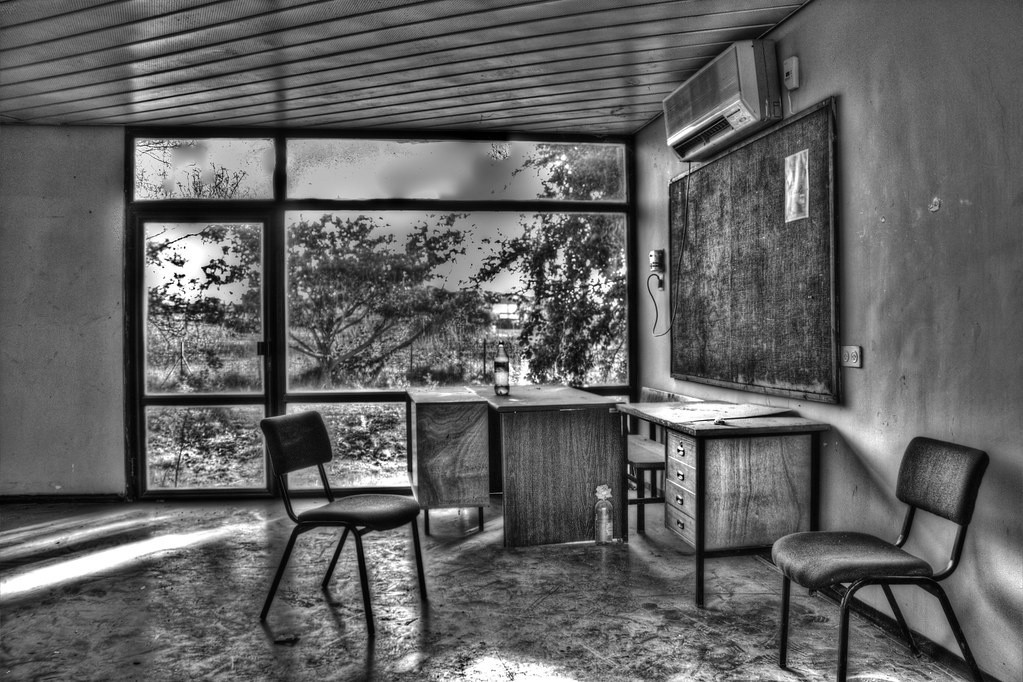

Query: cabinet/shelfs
[404,386,492,536]
[663,427,814,554]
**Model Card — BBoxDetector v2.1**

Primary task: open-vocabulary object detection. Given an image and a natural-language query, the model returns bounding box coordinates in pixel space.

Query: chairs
[771,436,991,682]
[257,410,428,636]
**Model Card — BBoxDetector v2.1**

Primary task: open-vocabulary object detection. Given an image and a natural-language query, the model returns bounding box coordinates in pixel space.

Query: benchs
[621,386,705,535]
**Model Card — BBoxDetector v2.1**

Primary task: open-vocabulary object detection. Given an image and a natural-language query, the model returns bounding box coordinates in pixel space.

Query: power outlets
[783,55,800,92]
[657,274,664,291]
[839,345,862,368]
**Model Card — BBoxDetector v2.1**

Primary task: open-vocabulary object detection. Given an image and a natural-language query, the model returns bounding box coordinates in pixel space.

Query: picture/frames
[665,94,842,405]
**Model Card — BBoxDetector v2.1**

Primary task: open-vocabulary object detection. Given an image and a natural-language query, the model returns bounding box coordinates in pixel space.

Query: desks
[616,398,832,611]
[464,382,628,552]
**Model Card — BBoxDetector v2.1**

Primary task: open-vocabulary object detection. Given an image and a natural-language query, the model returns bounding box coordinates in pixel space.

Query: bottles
[596,496,614,543]
[495,340,510,396]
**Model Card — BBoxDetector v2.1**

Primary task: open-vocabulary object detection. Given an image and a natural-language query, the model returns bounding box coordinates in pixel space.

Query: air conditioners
[661,39,783,164]
